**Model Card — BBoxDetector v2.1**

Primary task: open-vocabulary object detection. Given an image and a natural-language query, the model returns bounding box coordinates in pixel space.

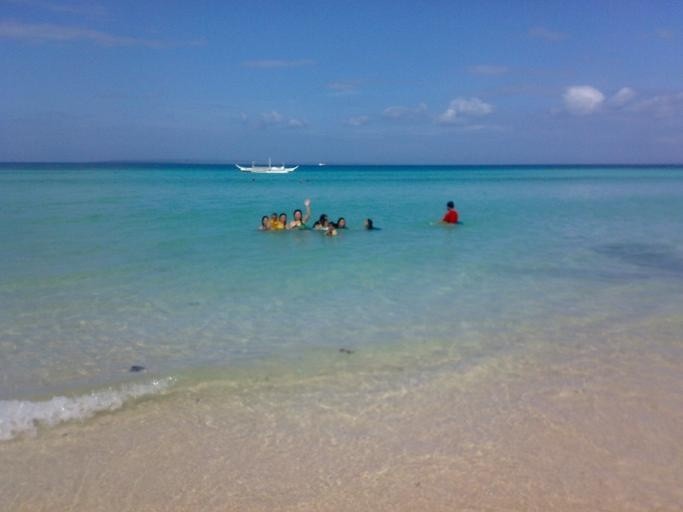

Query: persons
[364,218,373,230]
[257,197,350,237]
[437,200,459,225]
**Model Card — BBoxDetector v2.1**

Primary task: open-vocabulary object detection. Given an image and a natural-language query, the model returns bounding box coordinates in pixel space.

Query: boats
[317,162,326,167]
[232,155,300,174]
